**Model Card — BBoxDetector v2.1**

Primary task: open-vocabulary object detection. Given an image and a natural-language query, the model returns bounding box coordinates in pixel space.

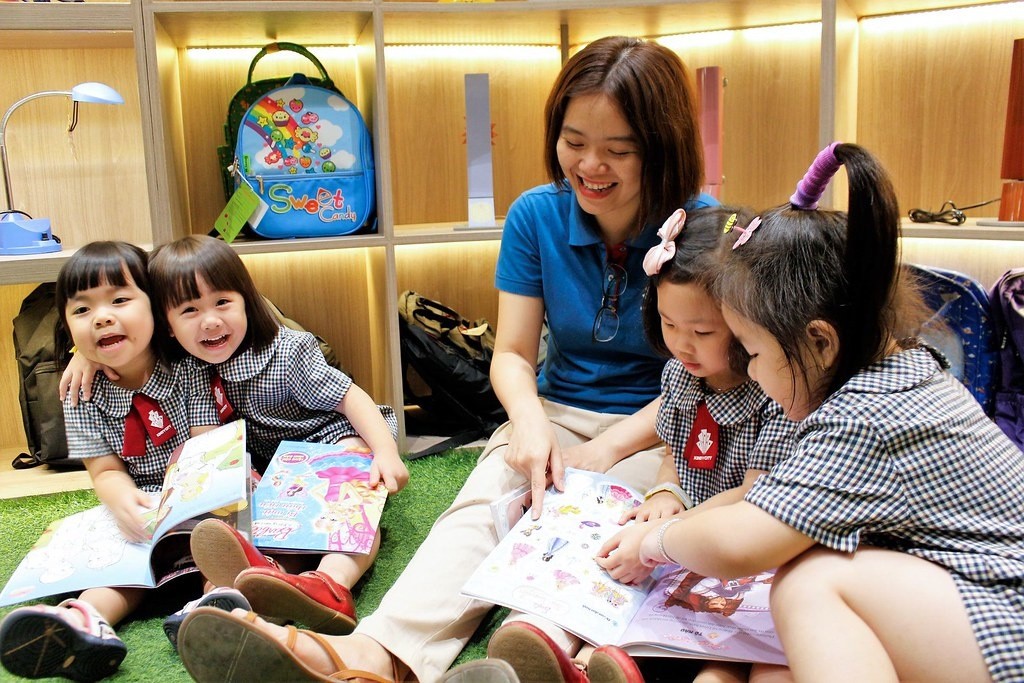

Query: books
[0,418,260,606]
[489,480,532,542]
[251,440,389,556]
[462,466,788,667]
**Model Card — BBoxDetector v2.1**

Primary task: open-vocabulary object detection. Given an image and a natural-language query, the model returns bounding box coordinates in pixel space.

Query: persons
[490,142,1024,683]
[180,36,721,683]
[0,234,408,683]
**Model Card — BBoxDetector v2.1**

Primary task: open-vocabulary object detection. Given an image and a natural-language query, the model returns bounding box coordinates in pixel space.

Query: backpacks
[901,262,1024,453]
[10,282,84,473]
[398,290,509,437]
[229,74,377,238]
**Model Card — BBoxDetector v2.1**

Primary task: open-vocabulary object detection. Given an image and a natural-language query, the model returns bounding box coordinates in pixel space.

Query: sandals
[431,657,521,683]
[178,606,402,683]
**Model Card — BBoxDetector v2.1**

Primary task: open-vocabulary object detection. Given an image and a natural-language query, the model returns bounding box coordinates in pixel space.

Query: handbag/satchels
[218,41,374,239]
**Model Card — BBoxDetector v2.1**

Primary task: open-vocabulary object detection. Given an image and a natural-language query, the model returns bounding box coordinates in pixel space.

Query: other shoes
[588,644,645,683]
[0,598,126,682]
[234,565,358,636]
[190,517,280,589]
[488,621,591,683]
[164,586,253,654]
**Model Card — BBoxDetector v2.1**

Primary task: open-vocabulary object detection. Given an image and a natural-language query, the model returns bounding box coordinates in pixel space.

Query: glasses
[591,263,627,344]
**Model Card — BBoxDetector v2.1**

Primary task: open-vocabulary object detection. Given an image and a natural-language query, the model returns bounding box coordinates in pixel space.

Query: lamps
[0,81,126,256]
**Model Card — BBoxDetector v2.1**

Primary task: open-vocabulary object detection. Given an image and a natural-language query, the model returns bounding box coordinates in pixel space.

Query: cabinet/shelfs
[0,1,1022,498]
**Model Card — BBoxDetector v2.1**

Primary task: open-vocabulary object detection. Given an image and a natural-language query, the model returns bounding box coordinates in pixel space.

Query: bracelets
[645,482,693,510]
[658,519,682,564]
